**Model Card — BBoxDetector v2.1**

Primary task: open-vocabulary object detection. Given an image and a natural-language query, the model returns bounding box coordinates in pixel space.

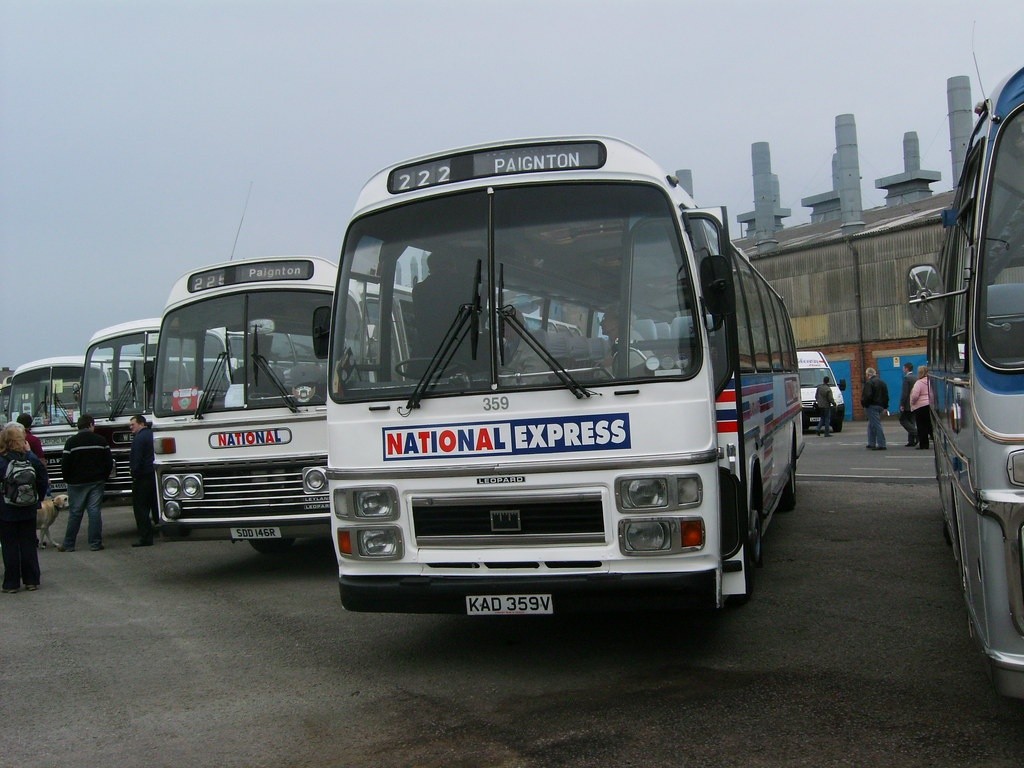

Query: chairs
[232,362,323,390]
[498,314,714,387]
[995,265,1024,284]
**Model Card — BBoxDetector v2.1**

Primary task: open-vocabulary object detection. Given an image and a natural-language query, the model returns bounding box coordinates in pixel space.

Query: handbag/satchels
[813,403,818,411]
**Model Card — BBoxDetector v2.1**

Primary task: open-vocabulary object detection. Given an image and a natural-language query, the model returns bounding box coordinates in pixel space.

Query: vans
[791,350,847,434]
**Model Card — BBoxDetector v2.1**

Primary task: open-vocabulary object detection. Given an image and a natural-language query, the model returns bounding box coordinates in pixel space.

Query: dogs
[36,495,70,550]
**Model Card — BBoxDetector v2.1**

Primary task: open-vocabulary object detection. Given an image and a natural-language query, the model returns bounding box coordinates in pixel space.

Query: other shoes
[866,445,876,448]
[928,436,934,440]
[25,583,36,591]
[872,446,886,450]
[91,544,104,550]
[815,429,820,436]
[917,447,929,449]
[57,545,75,552]
[905,442,916,446]
[824,434,832,437]
[131,540,154,547]
[0,586,18,593]
[916,433,919,445]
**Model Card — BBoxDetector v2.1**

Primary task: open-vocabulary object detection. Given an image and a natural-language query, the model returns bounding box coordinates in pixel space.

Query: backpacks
[0,450,38,505]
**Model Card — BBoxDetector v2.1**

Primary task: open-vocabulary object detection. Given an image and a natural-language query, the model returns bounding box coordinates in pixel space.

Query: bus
[0,354,326,512]
[310,131,807,621]
[902,60,1023,713]
[141,254,585,555]
[69,316,326,510]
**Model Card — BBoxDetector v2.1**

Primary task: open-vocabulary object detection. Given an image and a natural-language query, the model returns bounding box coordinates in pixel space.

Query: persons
[59,415,112,551]
[0,421,49,593]
[597,306,654,374]
[17,413,47,466]
[909,366,933,449]
[815,377,836,436]
[129,416,155,547]
[898,362,919,446]
[503,309,542,384]
[861,368,889,449]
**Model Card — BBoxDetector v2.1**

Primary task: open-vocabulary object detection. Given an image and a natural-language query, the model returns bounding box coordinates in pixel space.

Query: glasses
[602,314,618,320]
